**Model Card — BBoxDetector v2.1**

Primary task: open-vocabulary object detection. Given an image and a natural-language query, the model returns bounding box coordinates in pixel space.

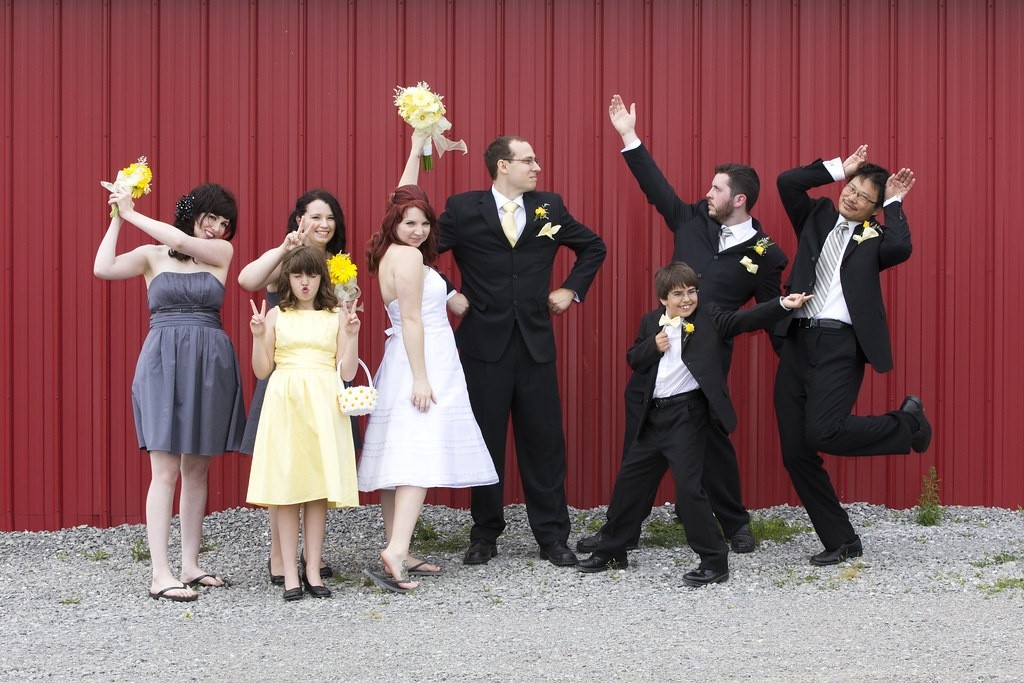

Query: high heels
[301,568,331,598]
[283,581,303,600]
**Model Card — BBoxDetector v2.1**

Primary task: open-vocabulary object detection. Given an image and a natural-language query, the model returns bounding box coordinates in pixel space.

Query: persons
[576,94,789,554]
[239,188,358,585]
[575,261,815,586]
[93,183,246,601]
[359,129,500,592]
[249,244,362,601]
[774,145,931,566]
[422,136,607,566]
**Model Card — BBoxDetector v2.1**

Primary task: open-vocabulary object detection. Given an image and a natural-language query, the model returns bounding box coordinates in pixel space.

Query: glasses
[844,184,877,204]
[668,289,700,298]
[503,158,541,166]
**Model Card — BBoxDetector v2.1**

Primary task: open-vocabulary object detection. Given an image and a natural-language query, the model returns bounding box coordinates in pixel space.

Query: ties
[501,202,520,248]
[718,228,733,253]
[801,222,849,319]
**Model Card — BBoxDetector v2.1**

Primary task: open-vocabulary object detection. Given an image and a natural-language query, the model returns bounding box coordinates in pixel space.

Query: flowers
[682,321,696,342]
[531,202,551,222]
[109,156,153,218]
[388,78,446,174]
[859,219,883,237]
[324,250,359,286]
[750,234,772,260]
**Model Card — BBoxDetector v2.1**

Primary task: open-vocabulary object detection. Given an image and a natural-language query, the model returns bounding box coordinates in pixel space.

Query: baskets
[338,357,376,417]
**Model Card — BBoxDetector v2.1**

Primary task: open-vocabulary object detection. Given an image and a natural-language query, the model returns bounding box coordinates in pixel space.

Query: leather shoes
[576,551,628,572]
[464,539,497,563]
[900,396,931,453]
[683,569,729,586]
[540,541,578,565]
[810,535,863,566]
[730,523,755,553]
[577,532,639,552]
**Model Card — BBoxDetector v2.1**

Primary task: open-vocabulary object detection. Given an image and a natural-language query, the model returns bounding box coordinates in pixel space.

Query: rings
[903,183,907,187]
[416,399,420,402]
[553,309,556,312]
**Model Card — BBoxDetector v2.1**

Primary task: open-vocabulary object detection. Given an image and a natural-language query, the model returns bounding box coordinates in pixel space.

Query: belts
[791,318,851,329]
[152,306,215,313]
[651,391,702,409]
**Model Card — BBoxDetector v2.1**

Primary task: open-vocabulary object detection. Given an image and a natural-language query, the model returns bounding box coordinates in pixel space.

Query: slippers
[363,569,419,594]
[383,560,444,578]
[181,573,225,587]
[149,584,200,601]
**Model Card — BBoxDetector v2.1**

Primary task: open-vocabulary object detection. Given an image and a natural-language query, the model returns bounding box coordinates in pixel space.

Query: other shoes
[300,548,333,577]
[267,560,285,584]
